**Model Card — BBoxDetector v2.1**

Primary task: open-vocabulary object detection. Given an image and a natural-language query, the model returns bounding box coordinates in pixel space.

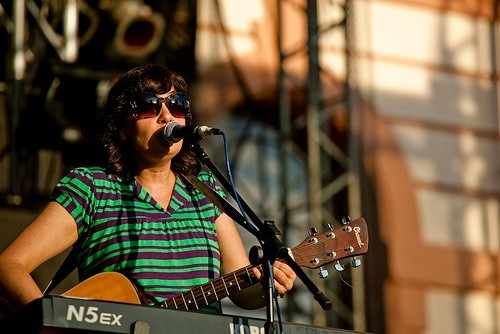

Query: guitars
[61,214,368,313]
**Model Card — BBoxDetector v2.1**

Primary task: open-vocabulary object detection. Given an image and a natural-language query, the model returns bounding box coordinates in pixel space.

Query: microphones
[162,122,225,144]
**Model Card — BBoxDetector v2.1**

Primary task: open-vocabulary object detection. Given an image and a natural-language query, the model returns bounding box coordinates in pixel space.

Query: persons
[1,64,297,310]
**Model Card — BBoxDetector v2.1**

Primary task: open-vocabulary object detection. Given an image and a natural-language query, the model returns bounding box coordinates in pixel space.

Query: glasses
[124,92,191,119]
